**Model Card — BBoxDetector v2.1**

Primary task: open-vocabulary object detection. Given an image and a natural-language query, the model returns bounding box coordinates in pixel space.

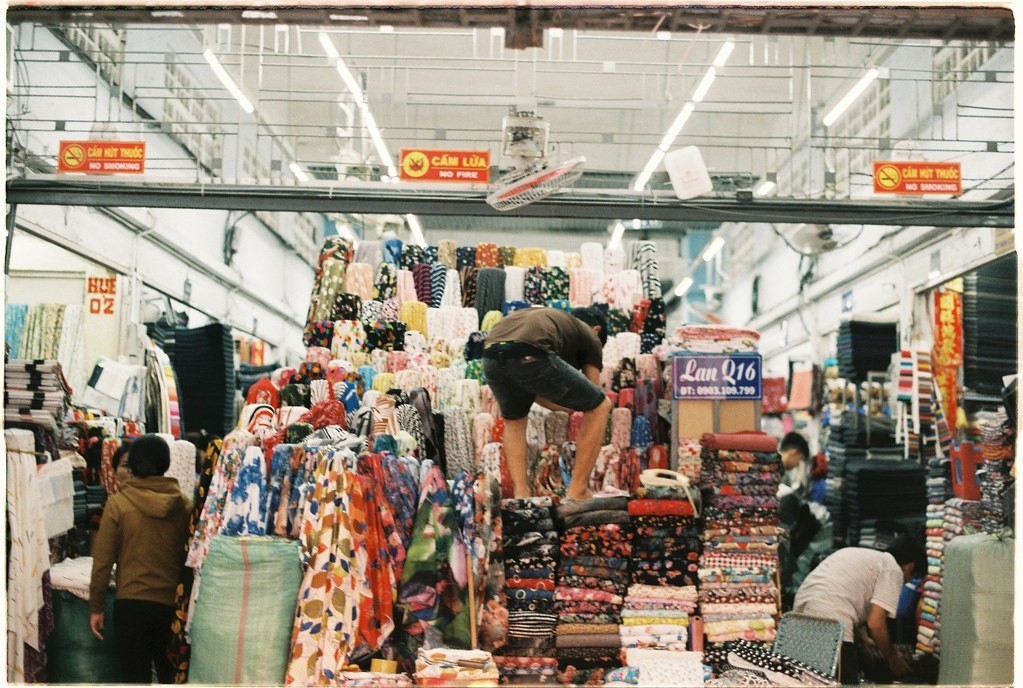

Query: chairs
[773,611,845,681]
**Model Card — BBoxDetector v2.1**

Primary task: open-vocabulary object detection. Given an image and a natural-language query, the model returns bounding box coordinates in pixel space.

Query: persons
[793,545,917,685]
[89,434,195,684]
[776,431,809,488]
[481,307,613,502]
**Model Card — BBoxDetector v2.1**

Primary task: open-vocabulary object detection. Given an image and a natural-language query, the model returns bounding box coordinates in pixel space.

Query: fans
[486,116,581,211]
